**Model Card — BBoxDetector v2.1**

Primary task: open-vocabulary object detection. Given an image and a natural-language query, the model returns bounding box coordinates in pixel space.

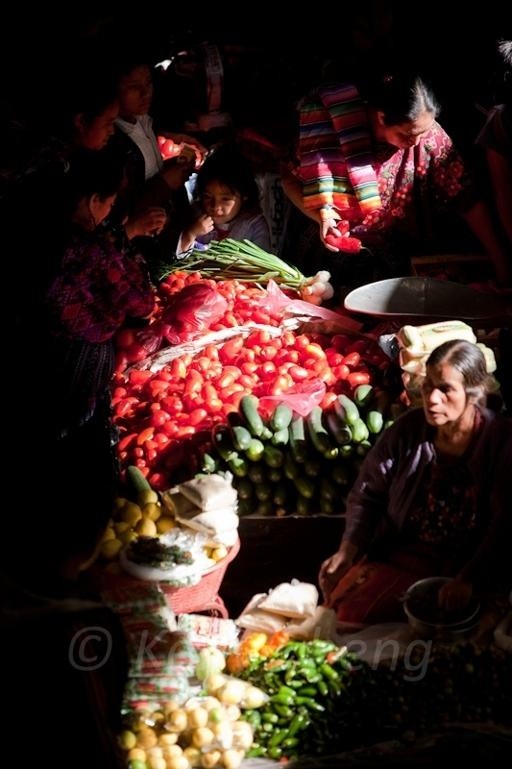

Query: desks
[279,178,320,257]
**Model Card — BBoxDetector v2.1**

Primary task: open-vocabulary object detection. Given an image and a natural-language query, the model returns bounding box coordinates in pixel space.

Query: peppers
[236,638,452,760]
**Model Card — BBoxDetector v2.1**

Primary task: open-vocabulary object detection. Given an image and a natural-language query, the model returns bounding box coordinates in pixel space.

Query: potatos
[94,491,227,571]
[117,674,265,768]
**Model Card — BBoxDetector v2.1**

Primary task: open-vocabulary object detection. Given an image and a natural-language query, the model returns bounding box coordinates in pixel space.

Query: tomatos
[109,270,394,486]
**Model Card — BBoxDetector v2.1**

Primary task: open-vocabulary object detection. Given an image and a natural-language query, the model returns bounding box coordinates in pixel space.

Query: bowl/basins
[100,537,240,614]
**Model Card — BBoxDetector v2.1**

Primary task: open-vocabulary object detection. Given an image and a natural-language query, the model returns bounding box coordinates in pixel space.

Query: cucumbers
[177,395,397,517]
[123,466,151,494]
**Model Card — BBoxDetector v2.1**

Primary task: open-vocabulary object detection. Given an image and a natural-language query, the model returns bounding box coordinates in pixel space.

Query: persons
[22,162,164,442]
[265,65,512,289]
[0,432,136,768]
[476,39,511,251]
[0,0,277,273]
[317,336,512,649]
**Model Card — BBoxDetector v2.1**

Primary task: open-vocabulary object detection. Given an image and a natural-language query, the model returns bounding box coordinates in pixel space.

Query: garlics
[302,271,334,307]
